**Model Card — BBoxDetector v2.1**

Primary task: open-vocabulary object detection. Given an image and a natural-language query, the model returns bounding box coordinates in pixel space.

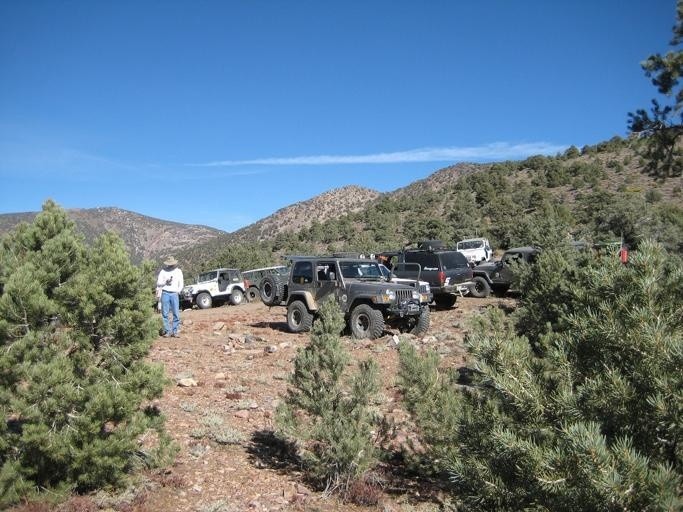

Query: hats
[163,256,178,266]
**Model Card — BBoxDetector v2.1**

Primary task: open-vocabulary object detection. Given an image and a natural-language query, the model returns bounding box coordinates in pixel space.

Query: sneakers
[163,332,171,337]
[172,334,180,339]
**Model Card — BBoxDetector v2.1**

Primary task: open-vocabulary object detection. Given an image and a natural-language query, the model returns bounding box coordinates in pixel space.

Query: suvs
[355,262,433,308]
[258,255,428,343]
[373,240,475,309]
[239,266,290,307]
[470,247,541,298]
[182,268,246,309]
[457,238,491,265]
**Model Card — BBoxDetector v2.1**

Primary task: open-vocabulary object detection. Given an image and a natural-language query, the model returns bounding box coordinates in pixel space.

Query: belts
[161,289,176,294]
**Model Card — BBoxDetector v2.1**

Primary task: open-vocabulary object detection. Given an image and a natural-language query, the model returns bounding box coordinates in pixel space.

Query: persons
[385,256,395,271]
[155,255,184,339]
